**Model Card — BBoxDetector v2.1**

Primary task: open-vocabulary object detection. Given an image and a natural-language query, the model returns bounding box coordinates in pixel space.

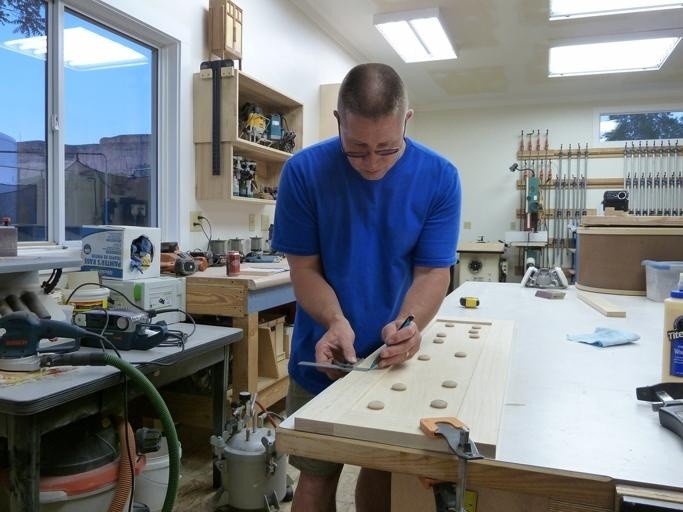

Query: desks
[184,250,294,451]
[0,319,245,511]
[273,280,683,508]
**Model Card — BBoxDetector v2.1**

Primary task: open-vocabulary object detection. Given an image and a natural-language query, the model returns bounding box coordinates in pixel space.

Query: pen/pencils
[369,315,414,369]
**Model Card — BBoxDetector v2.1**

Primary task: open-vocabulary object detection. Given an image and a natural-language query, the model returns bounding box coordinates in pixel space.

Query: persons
[270,61,462,512]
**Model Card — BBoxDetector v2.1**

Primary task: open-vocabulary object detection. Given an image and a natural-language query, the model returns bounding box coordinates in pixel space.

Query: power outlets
[190,210,204,232]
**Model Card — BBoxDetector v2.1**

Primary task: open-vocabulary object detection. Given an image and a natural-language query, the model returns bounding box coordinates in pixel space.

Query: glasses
[338,117,406,158]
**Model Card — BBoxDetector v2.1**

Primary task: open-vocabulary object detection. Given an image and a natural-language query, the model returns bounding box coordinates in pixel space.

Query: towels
[566,327,639,349]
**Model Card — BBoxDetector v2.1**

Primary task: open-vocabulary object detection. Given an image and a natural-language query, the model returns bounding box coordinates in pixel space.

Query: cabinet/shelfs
[190,69,303,206]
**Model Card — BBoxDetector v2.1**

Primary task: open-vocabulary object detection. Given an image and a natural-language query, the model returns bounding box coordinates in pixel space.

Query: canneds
[226,251,240,277]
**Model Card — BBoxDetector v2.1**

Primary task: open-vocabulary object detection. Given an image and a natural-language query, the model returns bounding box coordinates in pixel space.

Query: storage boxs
[642,260,683,303]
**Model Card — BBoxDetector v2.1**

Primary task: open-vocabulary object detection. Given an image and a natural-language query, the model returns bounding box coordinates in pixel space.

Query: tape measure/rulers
[298,361,369,371]
[460,297,479,307]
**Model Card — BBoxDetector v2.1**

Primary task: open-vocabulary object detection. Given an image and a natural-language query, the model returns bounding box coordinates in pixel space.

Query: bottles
[663,273,683,380]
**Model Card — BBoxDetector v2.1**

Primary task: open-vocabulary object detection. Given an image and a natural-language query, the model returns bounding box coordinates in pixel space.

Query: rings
[405,351,410,357]
